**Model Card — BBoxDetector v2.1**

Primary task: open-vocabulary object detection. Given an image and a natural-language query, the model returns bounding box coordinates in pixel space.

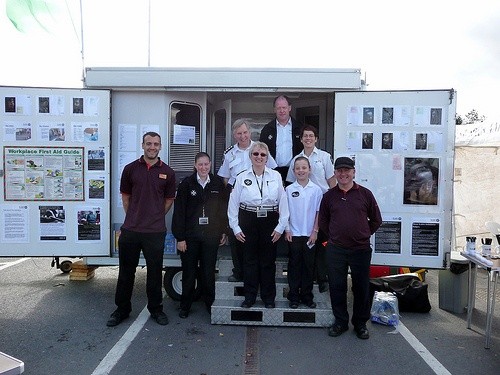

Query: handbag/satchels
[369,272,431,313]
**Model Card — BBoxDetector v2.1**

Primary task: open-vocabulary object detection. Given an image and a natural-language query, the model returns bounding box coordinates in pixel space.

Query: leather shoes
[329,323,349,336]
[353,325,369,339]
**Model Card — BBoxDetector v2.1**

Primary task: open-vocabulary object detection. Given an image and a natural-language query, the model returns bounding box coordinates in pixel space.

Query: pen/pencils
[267,181,268,185]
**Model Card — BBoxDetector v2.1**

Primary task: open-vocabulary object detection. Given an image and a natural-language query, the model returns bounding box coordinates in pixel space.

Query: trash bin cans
[438,251,477,314]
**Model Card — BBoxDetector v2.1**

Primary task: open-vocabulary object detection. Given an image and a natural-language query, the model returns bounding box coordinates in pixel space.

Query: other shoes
[179,306,190,318]
[265,303,275,308]
[106,309,130,326]
[151,312,169,325]
[307,302,316,308]
[289,302,299,309]
[228,274,244,282]
[241,302,255,307]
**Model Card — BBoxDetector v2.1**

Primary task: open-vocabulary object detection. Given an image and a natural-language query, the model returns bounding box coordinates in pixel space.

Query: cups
[466,241,475,252]
[482,244,491,257]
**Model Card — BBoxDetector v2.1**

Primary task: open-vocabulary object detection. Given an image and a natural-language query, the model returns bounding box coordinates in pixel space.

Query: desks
[460,251,500,350]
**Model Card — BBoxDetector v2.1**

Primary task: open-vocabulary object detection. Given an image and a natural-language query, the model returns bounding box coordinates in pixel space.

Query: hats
[334,157,355,169]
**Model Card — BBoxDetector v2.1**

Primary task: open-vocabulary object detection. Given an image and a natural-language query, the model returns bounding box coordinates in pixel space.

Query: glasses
[251,152,267,157]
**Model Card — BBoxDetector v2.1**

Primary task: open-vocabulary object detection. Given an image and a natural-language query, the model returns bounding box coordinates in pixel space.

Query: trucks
[0,65,461,304]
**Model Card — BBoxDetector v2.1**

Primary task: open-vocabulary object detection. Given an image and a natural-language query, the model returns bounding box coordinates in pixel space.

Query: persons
[217,119,278,282]
[285,126,336,293]
[171,152,228,319]
[382,133,393,149]
[78,211,100,224]
[105,132,175,326]
[259,95,305,258]
[227,142,288,308]
[88,150,104,159]
[282,157,322,309]
[318,157,382,339]
[362,133,373,149]
